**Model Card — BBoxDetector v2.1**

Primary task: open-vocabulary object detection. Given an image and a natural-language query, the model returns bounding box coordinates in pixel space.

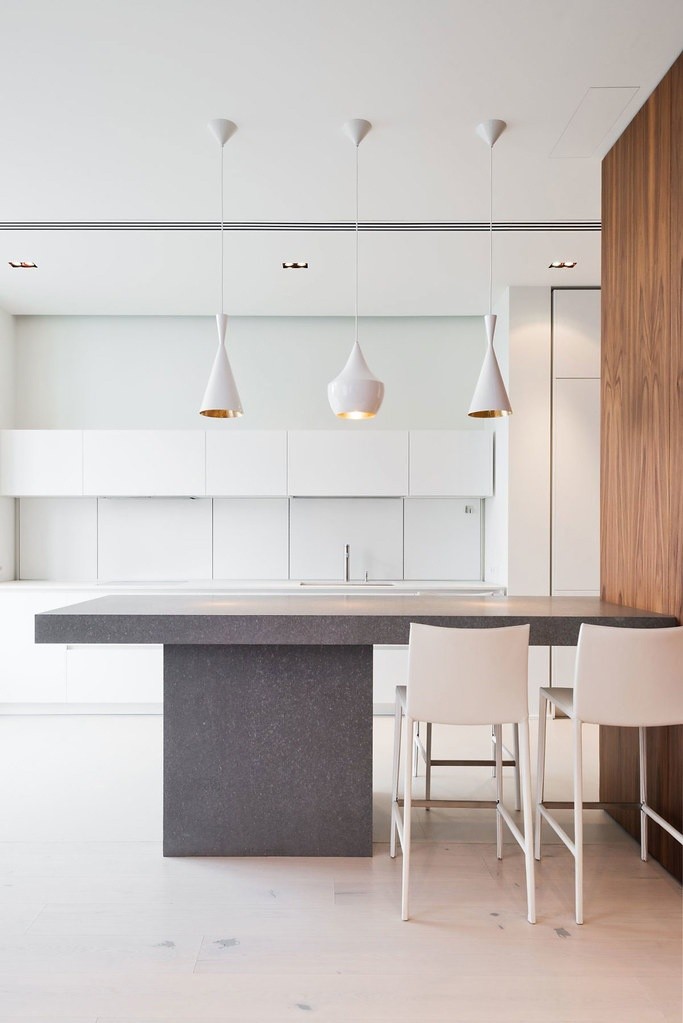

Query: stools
[534,622,683,923]
[389,622,536,923]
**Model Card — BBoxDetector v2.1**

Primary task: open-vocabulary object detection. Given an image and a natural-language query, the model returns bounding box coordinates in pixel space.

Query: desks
[35,595,677,857]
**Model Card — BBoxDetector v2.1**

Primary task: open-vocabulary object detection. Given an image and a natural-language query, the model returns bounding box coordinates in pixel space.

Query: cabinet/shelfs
[22,494,484,581]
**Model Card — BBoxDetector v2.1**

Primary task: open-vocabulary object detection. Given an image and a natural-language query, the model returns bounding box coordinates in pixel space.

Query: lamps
[467,116,517,421]
[327,117,383,417]
[198,118,246,420]
